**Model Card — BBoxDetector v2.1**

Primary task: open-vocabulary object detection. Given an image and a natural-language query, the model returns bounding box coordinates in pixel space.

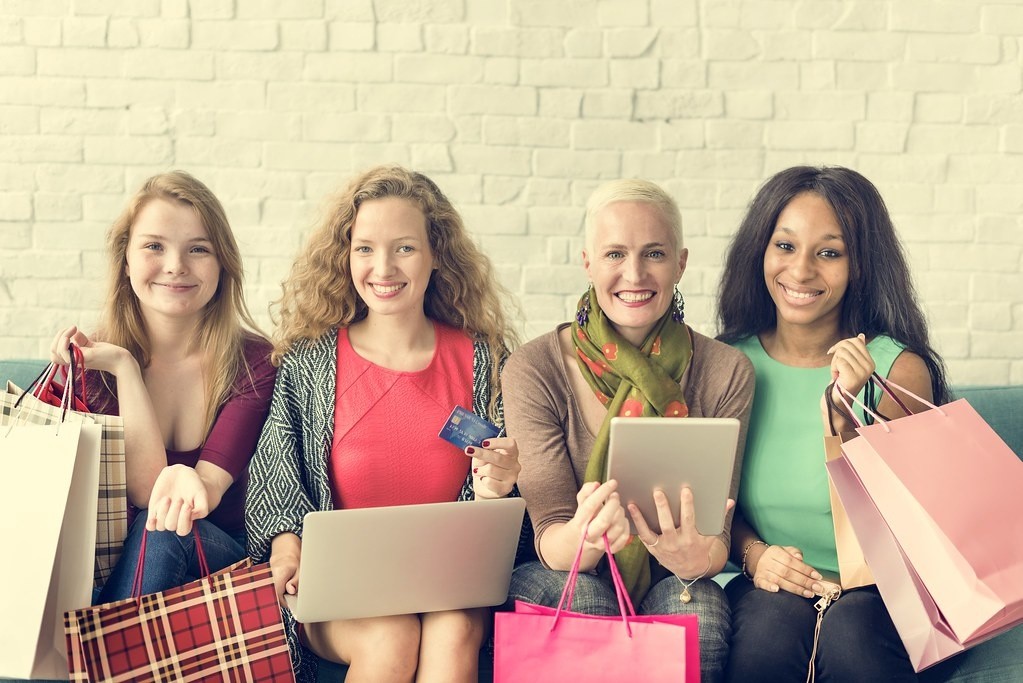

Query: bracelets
[673,552,712,604]
[742,539,770,582]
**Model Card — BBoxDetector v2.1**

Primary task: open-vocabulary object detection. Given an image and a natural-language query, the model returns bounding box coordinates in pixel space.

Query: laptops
[282,498,526,623]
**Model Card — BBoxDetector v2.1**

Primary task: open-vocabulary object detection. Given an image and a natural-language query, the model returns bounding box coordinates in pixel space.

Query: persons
[47,168,279,611]
[245,166,528,683]
[499,177,755,683]
[708,163,957,683]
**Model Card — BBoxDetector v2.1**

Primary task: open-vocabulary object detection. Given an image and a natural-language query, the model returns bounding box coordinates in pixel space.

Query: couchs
[0,364,1023,683]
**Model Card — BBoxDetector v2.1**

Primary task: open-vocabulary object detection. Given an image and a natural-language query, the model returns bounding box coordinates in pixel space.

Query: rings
[647,531,661,546]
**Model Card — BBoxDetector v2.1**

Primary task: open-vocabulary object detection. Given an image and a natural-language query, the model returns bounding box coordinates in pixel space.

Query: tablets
[606,418,740,536]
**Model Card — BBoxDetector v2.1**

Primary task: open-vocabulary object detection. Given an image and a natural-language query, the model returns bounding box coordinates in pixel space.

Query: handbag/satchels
[493,517,701,682]
[62,518,298,681]
[823,370,1021,673]
[1,342,131,680]
